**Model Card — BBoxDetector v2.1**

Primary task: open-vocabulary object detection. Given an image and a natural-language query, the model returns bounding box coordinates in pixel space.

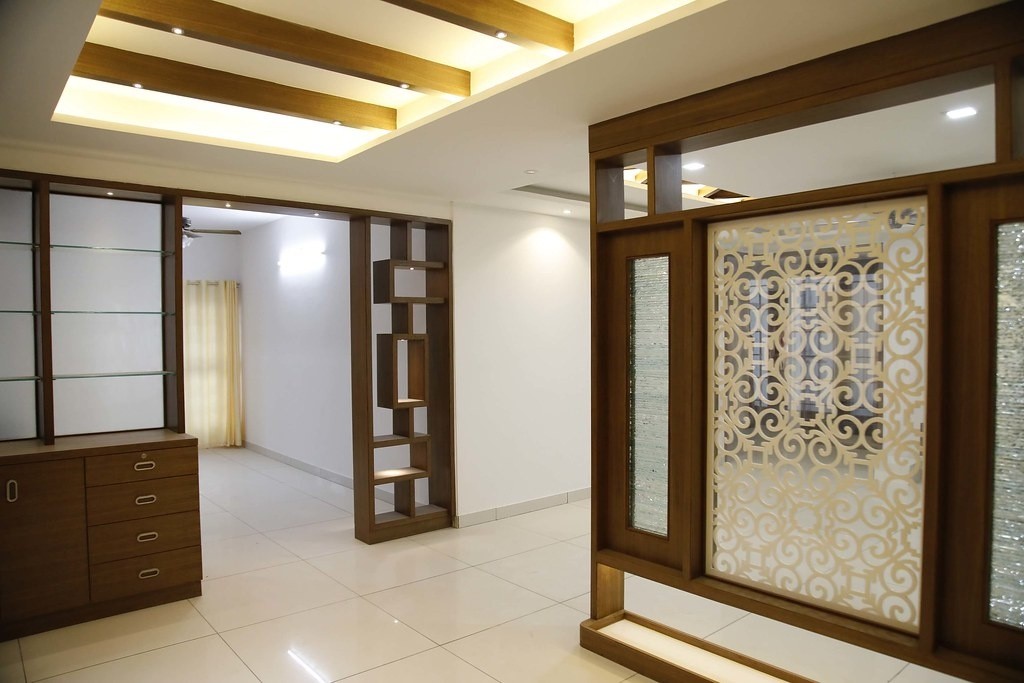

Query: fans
[182,216,242,238]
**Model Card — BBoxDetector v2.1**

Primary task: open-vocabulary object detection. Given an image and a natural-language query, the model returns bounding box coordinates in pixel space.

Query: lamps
[182,235,193,249]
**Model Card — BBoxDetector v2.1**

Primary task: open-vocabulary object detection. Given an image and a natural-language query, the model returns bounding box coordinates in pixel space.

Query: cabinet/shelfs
[0,430,203,643]
